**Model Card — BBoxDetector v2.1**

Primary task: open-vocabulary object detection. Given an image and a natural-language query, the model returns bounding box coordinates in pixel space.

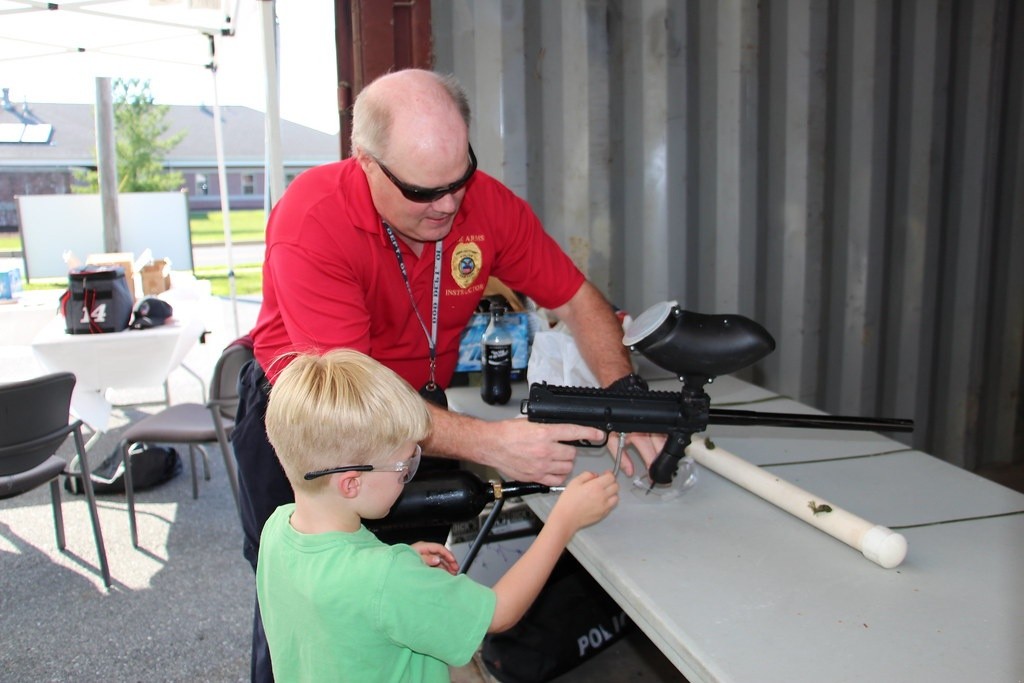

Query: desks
[444,370,1024,683]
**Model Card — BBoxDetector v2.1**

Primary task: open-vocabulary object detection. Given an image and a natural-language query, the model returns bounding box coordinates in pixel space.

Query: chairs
[0,371,114,590]
[118,342,256,549]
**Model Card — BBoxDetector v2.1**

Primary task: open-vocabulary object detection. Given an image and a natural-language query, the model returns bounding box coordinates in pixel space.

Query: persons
[255,348,619,683]
[232,68,669,683]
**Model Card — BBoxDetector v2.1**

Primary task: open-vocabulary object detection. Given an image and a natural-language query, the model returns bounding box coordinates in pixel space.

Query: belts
[253,359,272,398]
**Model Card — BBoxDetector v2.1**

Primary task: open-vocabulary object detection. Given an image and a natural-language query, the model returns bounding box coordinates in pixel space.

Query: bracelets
[609,374,649,388]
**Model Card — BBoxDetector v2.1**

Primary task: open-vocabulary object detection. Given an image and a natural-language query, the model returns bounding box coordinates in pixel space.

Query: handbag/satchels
[58,263,131,334]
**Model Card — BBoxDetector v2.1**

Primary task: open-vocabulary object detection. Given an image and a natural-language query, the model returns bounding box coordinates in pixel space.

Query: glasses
[370,140,478,204]
[304,444,423,485]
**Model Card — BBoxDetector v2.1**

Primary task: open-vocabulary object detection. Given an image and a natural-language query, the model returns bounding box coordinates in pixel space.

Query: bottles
[481,306,513,404]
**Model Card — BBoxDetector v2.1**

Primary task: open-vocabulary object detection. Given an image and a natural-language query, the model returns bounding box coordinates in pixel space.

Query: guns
[362,302,914,525]
